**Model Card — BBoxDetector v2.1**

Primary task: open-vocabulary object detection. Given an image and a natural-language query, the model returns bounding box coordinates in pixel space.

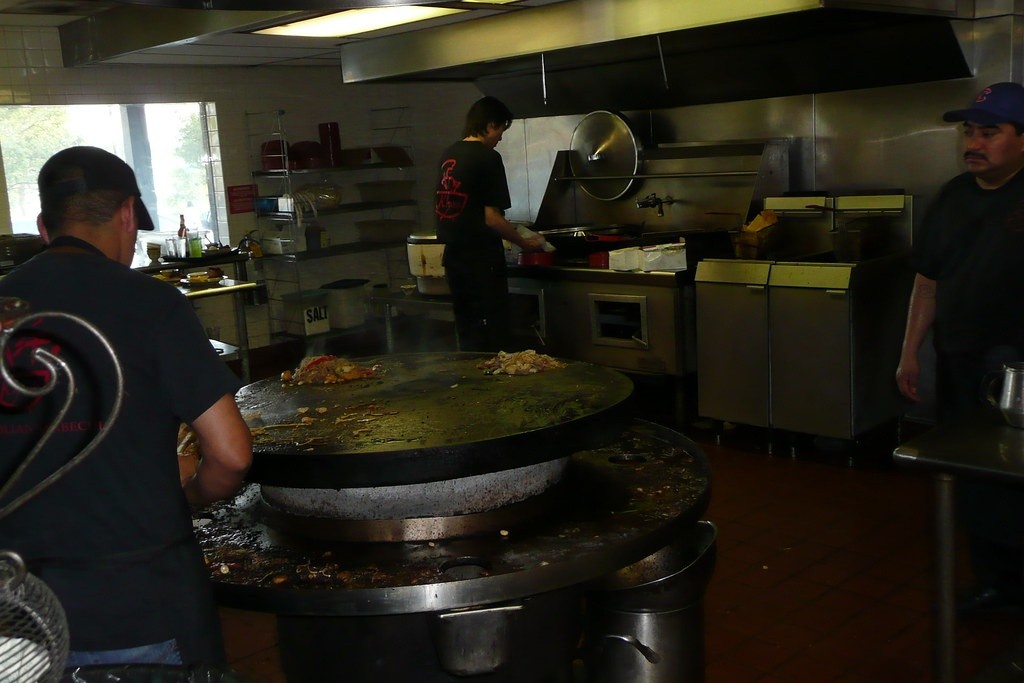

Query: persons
[896,82,1024,607]
[0,146,251,678]
[432,96,546,353]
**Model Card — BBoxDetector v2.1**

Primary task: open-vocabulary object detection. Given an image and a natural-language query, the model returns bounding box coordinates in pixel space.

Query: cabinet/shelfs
[247,105,430,353]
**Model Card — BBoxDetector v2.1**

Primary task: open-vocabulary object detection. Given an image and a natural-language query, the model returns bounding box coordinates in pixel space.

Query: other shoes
[958,588,1024,614]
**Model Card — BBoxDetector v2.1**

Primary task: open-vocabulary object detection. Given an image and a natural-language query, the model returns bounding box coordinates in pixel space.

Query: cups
[165,231,209,257]
[979,360,1024,430]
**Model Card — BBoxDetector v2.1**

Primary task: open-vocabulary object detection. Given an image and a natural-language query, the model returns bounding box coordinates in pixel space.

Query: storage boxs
[608,246,641,272]
[639,244,687,271]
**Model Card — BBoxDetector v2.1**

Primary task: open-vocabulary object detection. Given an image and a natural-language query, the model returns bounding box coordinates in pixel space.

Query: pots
[588,600,707,683]
[584,521,720,613]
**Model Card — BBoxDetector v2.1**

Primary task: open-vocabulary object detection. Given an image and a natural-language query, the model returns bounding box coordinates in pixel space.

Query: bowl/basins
[147,242,161,262]
[254,197,278,212]
[160,269,181,275]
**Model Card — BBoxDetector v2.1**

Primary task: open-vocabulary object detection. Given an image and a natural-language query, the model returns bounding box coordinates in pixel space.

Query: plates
[181,275,224,286]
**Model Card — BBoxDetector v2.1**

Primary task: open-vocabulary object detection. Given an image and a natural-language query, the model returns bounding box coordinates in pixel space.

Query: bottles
[177,214,190,256]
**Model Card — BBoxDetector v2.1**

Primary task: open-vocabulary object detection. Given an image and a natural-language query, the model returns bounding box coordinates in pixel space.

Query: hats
[943,82,1024,125]
[39,147,154,230]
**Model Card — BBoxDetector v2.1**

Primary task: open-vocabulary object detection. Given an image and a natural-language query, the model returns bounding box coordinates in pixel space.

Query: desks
[370,290,461,355]
[175,278,266,386]
[892,409,1023,683]
